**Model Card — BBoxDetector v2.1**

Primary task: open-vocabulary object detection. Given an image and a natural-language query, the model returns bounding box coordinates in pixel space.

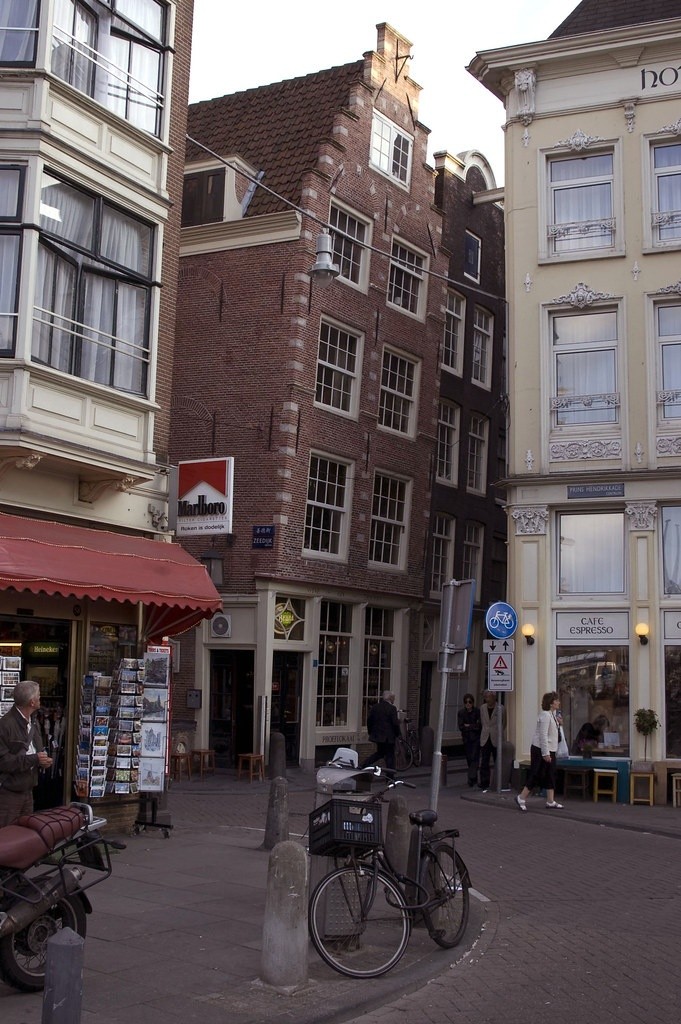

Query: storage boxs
[309,799,382,854]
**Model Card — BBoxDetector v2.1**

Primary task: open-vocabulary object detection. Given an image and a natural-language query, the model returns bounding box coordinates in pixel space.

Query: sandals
[514,795,527,811]
[545,801,564,809]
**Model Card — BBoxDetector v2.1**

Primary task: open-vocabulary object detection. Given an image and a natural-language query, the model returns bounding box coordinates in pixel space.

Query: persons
[515,692,564,812]
[572,715,609,757]
[458,694,482,787]
[0,681,52,828]
[479,689,507,789]
[363,691,400,785]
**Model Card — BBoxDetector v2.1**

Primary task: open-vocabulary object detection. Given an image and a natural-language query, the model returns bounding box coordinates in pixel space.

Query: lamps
[307,227,339,289]
[200,531,236,587]
[636,623,649,645]
[521,623,534,645]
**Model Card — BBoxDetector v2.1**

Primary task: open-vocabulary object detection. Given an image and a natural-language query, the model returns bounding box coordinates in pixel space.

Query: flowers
[578,739,597,751]
[633,707,662,735]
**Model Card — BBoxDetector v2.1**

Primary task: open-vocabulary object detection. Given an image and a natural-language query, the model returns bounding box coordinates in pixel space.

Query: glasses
[464,700,473,704]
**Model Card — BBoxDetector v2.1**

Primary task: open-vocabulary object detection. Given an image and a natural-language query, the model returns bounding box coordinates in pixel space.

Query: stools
[563,766,592,799]
[238,753,262,783]
[520,760,531,790]
[629,769,655,807]
[190,748,216,777]
[671,772,681,808]
[171,753,191,782]
[594,768,617,804]
[539,765,566,793]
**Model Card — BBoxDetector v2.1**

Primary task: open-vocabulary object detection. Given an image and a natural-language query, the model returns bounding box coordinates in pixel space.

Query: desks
[581,747,625,755]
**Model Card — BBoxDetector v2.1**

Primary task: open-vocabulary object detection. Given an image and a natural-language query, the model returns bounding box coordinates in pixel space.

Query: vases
[583,750,592,759]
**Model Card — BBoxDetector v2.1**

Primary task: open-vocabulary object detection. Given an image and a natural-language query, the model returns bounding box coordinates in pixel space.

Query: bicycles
[395,708,422,771]
[308,780,473,977]
[328,749,397,781]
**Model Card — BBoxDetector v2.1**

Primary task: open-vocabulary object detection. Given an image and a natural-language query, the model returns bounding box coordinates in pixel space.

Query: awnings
[0,513,223,644]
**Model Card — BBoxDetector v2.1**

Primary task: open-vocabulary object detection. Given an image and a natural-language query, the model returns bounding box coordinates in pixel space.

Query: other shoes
[476,783,488,790]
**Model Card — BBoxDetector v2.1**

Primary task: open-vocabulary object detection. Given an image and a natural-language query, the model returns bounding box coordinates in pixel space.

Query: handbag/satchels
[555,725,570,759]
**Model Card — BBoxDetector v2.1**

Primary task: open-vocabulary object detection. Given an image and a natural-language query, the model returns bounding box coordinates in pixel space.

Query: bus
[560,662,618,698]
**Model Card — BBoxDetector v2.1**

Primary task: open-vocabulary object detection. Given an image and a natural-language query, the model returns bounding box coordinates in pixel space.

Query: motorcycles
[0,798,129,993]
